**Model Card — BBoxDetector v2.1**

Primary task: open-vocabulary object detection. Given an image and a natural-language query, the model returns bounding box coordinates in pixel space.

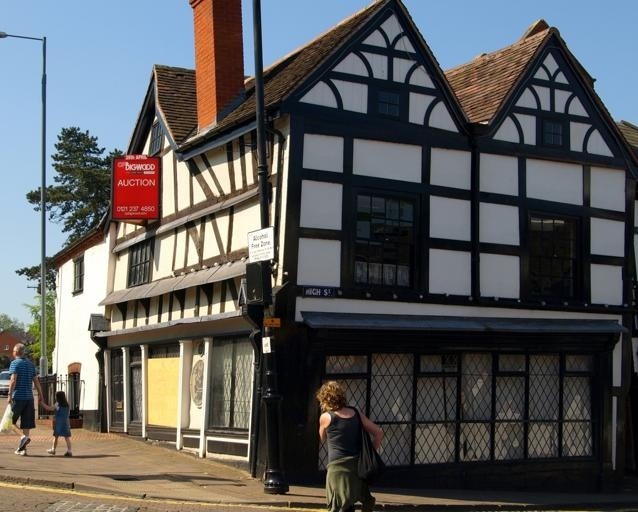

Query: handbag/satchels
[356,427,385,480]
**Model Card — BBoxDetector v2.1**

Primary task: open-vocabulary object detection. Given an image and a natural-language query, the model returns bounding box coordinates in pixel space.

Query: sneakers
[14,449,26,456]
[17,436,31,451]
[47,449,56,455]
[63,452,72,457]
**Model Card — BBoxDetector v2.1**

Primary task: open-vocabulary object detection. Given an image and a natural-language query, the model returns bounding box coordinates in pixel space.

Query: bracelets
[7,392,12,395]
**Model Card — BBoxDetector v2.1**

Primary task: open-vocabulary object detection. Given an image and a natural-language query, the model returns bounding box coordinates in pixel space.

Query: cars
[0,370,11,397]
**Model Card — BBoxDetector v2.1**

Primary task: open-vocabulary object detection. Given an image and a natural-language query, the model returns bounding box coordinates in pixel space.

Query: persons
[6,342,44,458]
[314,379,385,512]
[36,390,73,458]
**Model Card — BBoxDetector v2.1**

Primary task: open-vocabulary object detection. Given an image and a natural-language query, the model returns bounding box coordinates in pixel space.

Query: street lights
[0,31,48,378]
